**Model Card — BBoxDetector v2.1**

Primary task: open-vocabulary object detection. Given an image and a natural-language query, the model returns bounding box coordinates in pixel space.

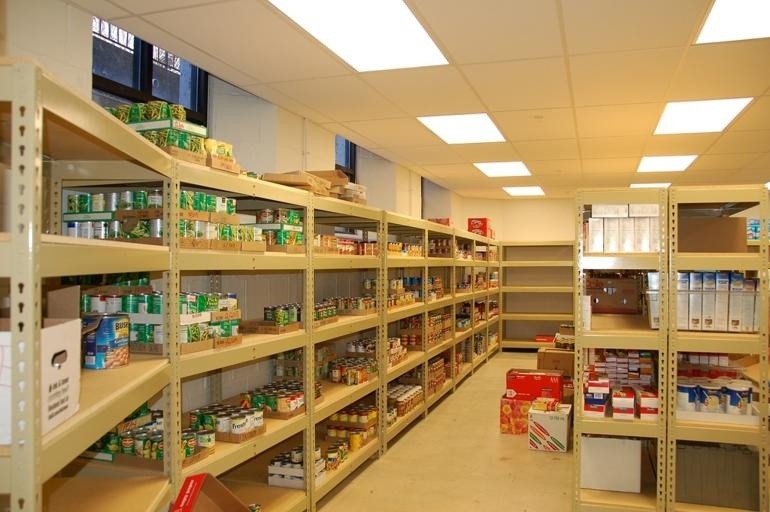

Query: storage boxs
[500,323,576,453]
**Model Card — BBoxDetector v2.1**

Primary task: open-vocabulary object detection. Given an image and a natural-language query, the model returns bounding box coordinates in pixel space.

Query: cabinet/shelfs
[668,184,769,509]
[0,52,499,512]
[497,240,577,352]
[574,185,667,509]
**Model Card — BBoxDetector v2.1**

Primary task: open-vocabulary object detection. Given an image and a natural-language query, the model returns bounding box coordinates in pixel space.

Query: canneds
[80,271,238,369]
[276,327,422,385]
[190,401,265,443]
[93,399,215,461]
[240,378,321,413]
[66,101,259,180]
[270,385,423,480]
[263,276,422,327]
[260,208,423,256]
[66,190,262,241]
